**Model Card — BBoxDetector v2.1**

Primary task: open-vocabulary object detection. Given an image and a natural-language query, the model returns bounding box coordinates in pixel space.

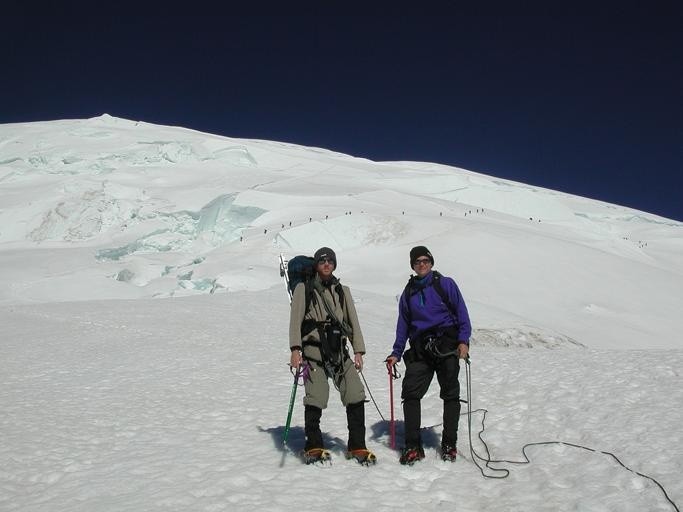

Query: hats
[313,247,337,269]
[410,246,434,269]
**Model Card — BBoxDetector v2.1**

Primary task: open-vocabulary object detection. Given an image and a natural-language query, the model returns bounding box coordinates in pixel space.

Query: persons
[402,211,405,215]
[623,237,647,248]
[239,210,352,242]
[440,211,442,216]
[528,216,532,223]
[539,219,542,224]
[288,246,379,465]
[385,245,473,465]
[465,207,484,217]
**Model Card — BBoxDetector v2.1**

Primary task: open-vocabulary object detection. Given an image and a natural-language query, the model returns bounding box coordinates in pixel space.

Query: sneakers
[304,446,331,465]
[400,448,425,466]
[347,449,376,466]
[441,445,456,462]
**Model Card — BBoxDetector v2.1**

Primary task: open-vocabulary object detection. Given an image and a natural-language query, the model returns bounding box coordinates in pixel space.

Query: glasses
[319,259,334,265]
[415,259,431,264]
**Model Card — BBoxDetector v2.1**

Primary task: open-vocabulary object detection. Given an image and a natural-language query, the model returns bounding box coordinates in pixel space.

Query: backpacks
[288,255,344,312]
